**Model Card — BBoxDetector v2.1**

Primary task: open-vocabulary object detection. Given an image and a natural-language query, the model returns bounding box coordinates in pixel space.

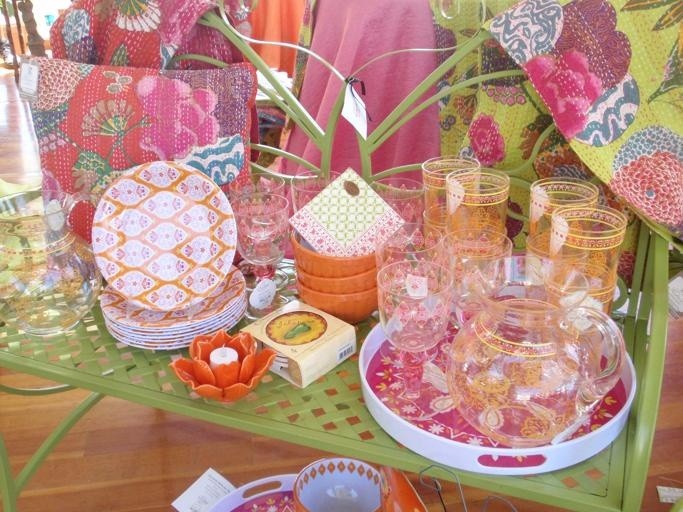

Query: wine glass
[231,173,289,294]
[228,192,289,319]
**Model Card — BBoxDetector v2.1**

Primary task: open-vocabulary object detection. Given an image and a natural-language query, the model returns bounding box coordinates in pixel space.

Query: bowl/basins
[290,230,407,323]
[294,456,390,511]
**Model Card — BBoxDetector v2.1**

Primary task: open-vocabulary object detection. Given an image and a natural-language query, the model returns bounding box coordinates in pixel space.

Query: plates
[91,160,248,352]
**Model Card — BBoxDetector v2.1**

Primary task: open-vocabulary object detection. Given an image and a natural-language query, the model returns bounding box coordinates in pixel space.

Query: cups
[421,153,479,261]
[376,260,455,354]
[375,223,447,271]
[442,230,512,309]
[445,167,510,255]
[524,175,600,300]
[546,203,628,313]
[371,176,426,245]
[291,169,342,214]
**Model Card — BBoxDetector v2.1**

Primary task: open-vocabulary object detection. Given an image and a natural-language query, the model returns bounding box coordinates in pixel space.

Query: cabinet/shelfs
[0,187,668,512]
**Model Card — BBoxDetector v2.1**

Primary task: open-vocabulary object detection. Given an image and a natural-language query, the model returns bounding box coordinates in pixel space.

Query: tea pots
[447,287,627,449]
[0,190,101,338]
